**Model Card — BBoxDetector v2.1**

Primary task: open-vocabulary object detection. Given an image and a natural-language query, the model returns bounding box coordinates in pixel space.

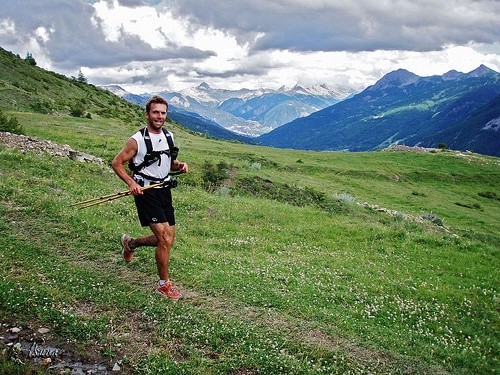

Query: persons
[112,96,188,300]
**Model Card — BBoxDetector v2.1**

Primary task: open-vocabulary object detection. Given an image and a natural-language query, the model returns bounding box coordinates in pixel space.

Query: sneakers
[155,281,183,300]
[121,234,135,261]
